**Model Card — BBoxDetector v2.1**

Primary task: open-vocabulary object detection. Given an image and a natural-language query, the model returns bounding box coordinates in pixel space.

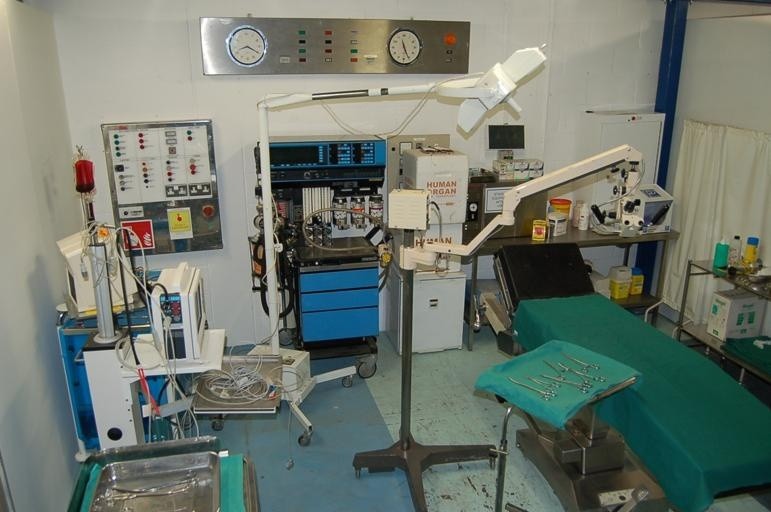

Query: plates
[86,451,221,512]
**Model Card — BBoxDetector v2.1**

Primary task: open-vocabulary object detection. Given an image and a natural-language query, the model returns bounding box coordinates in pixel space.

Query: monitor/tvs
[487,124,526,159]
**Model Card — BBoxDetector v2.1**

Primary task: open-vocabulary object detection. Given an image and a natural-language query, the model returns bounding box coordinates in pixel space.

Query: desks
[463,216,679,352]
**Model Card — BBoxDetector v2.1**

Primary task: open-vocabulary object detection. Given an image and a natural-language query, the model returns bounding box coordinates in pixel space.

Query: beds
[493,242,771,508]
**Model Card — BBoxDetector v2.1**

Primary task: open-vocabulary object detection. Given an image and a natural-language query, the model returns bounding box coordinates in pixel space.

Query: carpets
[77,344,417,510]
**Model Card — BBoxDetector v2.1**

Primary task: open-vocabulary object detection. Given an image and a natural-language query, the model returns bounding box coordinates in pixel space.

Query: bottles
[577,204,590,231]
[743,237,759,264]
[713,235,730,268]
[728,236,742,267]
